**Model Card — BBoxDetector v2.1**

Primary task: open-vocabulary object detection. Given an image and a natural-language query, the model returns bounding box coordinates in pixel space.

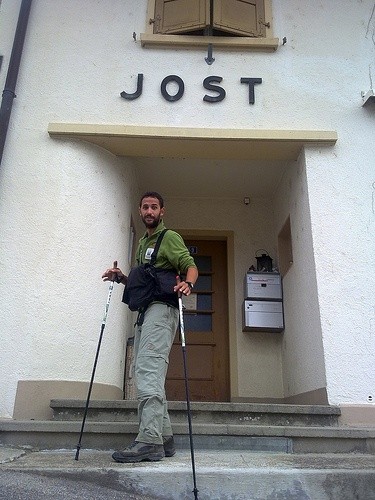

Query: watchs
[185,282,194,288]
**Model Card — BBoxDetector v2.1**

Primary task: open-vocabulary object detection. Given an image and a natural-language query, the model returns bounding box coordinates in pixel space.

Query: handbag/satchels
[122,230,169,309]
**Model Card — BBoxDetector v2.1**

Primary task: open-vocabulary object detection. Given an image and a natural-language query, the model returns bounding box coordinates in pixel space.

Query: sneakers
[112,436,175,463]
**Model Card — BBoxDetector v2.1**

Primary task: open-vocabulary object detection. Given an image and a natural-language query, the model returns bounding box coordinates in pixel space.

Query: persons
[101,192,198,462]
